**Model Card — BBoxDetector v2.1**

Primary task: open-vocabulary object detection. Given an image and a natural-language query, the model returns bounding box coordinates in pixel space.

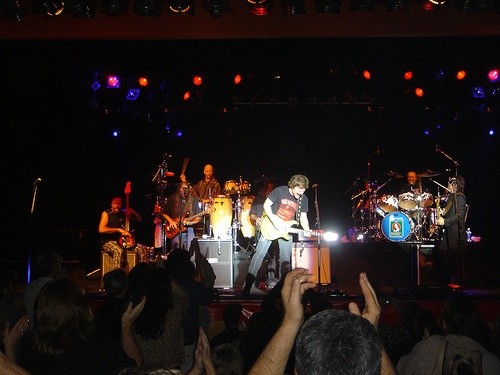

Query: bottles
[466,228,472,242]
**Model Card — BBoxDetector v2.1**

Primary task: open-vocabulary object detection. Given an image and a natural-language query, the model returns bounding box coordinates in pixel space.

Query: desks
[292,239,332,286]
[331,240,420,300]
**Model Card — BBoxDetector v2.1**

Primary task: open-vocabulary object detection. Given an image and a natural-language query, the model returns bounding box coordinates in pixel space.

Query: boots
[242,273,256,298]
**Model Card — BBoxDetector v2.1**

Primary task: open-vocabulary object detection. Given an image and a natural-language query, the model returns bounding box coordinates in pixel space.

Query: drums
[399,192,417,210]
[416,193,433,207]
[377,193,399,212]
[209,194,232,238]
[224,180,238,194]
[240,196,256,238]
[242,180,251,194]
[381,210,413,242]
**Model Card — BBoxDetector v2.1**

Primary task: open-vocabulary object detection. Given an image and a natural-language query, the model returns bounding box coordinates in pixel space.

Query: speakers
[190,239,234,290]
[410,245,454,289]
[100,251,139,291]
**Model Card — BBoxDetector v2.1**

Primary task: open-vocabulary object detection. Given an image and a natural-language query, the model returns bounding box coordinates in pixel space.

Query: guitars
[261,213,338,242]
[118,182,135,249]
[435,196,444,240]
[164,208,217,239]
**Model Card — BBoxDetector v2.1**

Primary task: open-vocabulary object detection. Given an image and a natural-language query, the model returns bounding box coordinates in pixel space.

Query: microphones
[34,178,41,184]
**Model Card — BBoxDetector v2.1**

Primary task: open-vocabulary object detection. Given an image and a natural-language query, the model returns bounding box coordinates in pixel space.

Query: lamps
[0,0,500,21]
[401,66,500,101]
[91,72,140,101]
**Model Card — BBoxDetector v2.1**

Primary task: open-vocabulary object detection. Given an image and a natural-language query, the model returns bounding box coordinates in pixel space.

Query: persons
[125,261,186,375]
[210,303,244,375]
[191,164,221,200]
[395,271,500,375]
[433,175,467,300]
[22,247,68,326]
[14,278,147,375]
[163,238,217,375]
[401,171,427,193]
[95,269,131,346]
[99,197,147,269]
[241,174,312,296]
[247,268,398,375]
[160,182,202,248]
[249,180,278,289]
[0,315,30,375]
